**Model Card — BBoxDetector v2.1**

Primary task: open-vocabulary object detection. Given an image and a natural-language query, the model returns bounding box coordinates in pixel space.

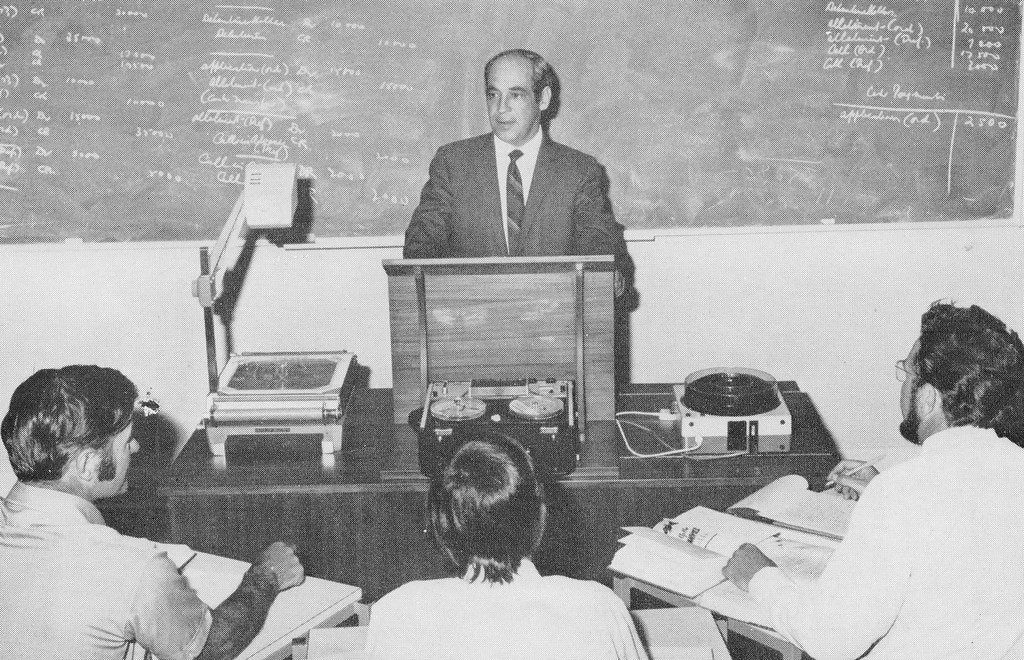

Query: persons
[0,365,303,660]
[402,48,626,301]
[367,430,651,660]
[722,302,1024,660]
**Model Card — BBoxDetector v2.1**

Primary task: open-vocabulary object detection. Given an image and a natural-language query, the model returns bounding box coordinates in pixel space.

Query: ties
[505,149,526,256]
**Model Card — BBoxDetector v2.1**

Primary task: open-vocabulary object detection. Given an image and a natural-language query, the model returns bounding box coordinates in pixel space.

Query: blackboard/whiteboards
[1,0,1021,258]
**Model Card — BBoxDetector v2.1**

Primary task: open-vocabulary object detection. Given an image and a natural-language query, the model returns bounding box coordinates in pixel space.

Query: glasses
[895,360,918,383]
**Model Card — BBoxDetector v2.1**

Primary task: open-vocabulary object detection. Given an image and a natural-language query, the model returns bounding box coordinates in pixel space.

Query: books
[154,544,196,567]
[608,506,777,599]
[728,474,859,536]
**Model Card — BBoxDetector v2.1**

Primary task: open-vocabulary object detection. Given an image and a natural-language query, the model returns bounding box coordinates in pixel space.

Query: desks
[155,381,843,660]
[614,574,805,660]
[155,544,363,660]
[291,606,732,660]
